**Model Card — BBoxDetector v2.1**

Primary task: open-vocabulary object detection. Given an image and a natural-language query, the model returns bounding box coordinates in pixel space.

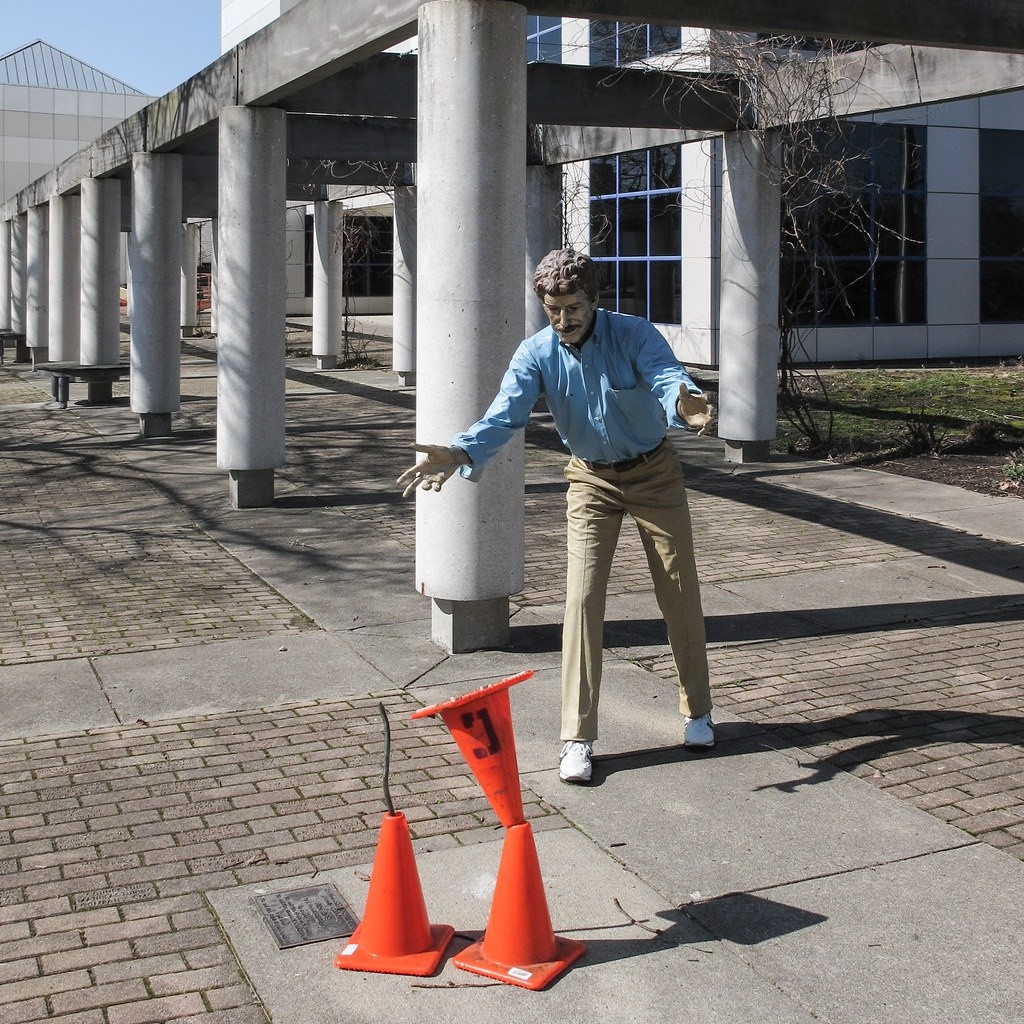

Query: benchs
[35,359,129,410]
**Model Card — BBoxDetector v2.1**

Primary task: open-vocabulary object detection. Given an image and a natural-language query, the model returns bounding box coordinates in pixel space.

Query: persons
[395,249,714,782]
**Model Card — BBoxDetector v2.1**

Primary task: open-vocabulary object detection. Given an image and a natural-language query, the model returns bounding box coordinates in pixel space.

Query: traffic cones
[410,670,553,827]
[452,824,584,989]
[337,811,452,974]
